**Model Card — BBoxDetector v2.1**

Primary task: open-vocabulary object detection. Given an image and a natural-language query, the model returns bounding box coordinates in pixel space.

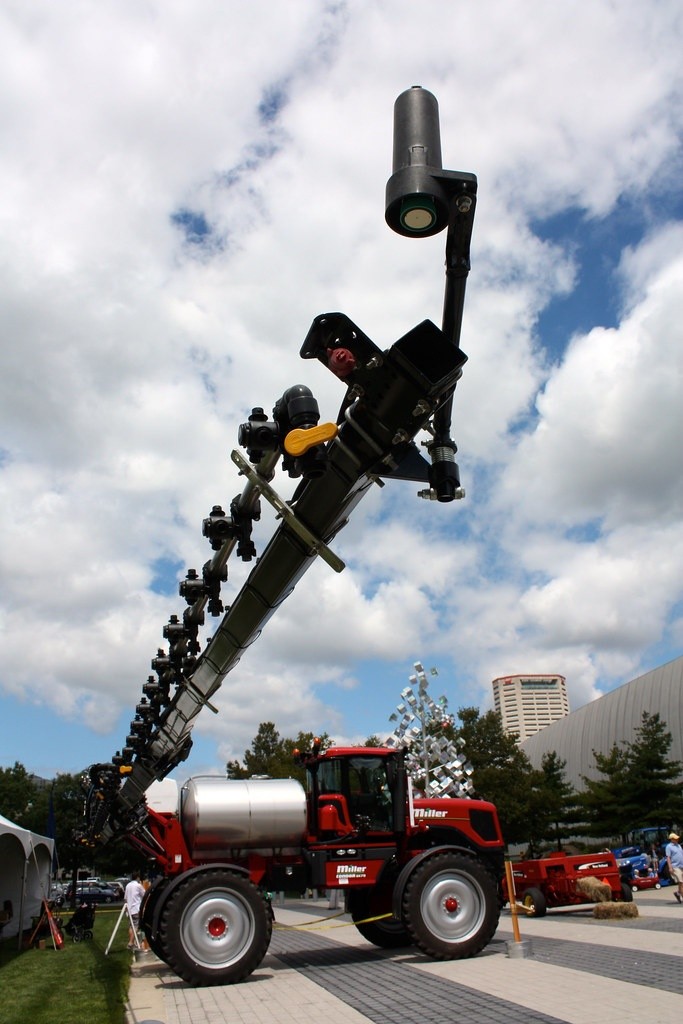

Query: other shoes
[674,892,683,903]
[127,944,137,949]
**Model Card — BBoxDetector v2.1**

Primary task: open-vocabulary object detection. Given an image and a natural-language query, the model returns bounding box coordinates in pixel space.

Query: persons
[124,871,152,952]
[54,889,97,930]
[307,889,314,899]
[664,832,683,904]
[266,891,272,901]
[630,843,662,880]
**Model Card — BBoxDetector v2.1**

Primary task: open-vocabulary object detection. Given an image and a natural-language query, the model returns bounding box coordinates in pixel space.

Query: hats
[669,833,680,839]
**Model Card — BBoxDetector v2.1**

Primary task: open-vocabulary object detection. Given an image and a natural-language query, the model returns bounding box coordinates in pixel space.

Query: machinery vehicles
[60,86,506,985]
[502,827,675,917]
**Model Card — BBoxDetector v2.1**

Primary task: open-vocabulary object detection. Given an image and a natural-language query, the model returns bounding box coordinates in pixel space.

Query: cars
[50,877,131,904]
